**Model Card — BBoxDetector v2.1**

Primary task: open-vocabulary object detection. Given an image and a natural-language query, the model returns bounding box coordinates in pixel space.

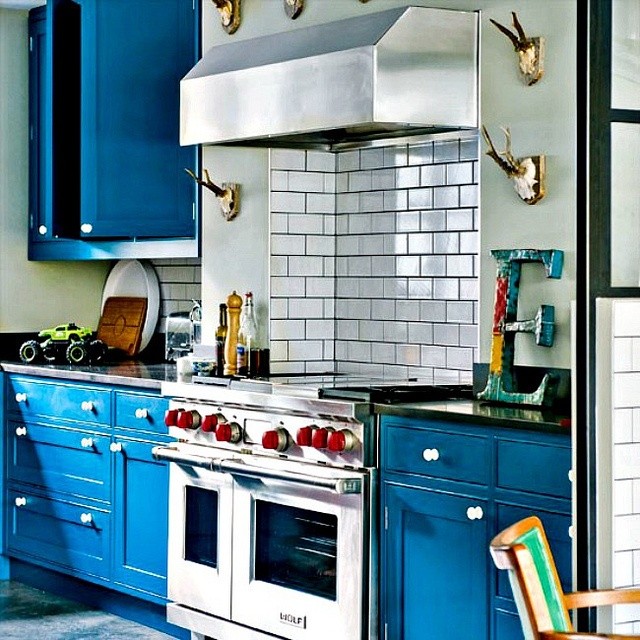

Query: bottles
[214,303,229,375]
[236,292,259,378]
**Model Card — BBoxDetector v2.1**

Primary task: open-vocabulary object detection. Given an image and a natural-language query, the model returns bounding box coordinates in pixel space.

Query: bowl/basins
[192,359,216,377]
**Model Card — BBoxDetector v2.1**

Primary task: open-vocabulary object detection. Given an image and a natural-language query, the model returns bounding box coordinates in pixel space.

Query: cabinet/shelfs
[114,389,172,606]
[3,372,111,582]
[383,414,491,640]
[27,0,201,260]
[492,428,571,639]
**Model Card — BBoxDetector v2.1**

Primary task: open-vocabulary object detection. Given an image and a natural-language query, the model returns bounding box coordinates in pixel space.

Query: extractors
[179,5,479,155]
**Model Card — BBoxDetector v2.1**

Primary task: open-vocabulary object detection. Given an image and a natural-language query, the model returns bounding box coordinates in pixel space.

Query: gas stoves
[228,371,473,402]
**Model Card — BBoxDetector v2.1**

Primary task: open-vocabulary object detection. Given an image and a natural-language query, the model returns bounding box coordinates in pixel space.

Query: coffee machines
[164,312,194,364]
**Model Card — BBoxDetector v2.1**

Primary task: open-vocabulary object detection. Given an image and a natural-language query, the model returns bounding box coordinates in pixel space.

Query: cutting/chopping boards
[95,296,148,357]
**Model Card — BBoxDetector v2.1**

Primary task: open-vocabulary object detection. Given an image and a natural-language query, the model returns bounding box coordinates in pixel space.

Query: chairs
[488,513,640,640]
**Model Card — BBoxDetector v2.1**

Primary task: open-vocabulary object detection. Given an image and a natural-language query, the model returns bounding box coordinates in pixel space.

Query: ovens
[151,381,378,639]
